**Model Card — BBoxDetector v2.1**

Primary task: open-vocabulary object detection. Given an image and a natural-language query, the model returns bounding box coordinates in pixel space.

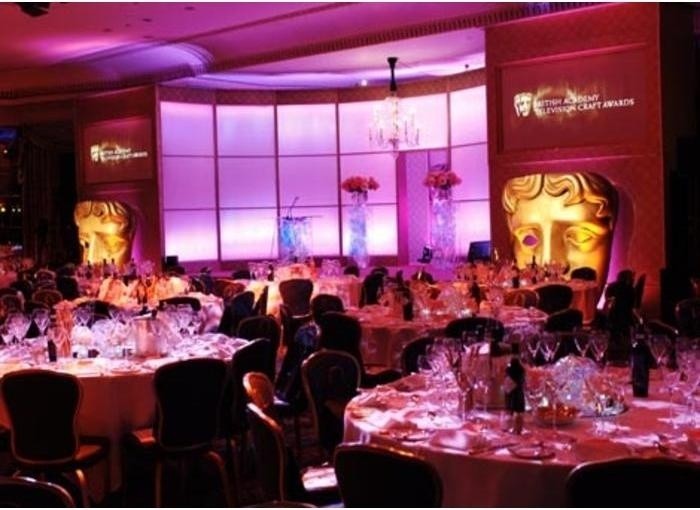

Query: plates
[507,442,556,458]
[109,362,141,373]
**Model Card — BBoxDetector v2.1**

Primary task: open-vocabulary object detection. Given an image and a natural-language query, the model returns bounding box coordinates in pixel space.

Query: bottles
[395,273,412,320]
[511,259,519,288]
[631,328,649,398]
[531,256,537,284]
[268,263,274,281]
[470,274,481,300]
[505,342,525,412]
[48,309,56,361]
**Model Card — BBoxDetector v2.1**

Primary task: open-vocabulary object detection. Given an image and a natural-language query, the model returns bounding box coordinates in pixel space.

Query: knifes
[468,442,520,456]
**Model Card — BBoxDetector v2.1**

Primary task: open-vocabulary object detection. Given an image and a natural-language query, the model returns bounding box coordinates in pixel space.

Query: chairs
[358,271,383,306]
[238,315,279,390]
[215,290,254,342]
[319,310,402,388]
[71,312,108,358]
[56,274,80,300]
[124,356,230,508]
[301,350,360,456]
[534,309,583,366]
[71,300,131,322]
[570,267,597,281]
[401,335,433,379]
[278,279,313,315]
[230,339,279,456]
[244,372,341,496]
[25,302,50,337]
[335,442,442,508]
[274,325,319,446]
[274,303,316,400]
[445,318,504,343]
[244,402,317,508]
[534,285,574,316]
[0,369,111,508]
[152,296,207,334]
[564,456,700,509]
[253,285,268,314]
[505,289,538,308]
[33,290,62,305]
[2,296,21,317]
[312,293,344,328]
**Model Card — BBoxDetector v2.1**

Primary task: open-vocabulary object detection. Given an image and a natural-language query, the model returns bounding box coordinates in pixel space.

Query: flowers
[342,175,380,206]
[422,163,462,199]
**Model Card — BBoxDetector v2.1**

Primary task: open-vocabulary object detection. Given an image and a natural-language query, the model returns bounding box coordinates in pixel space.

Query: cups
[584,372,614,429]
[544,336,561,363]
[108,309,120,321]
[319,259,342,279]
[79,307,89,327]
[526,391,539,424]
[177,304,192,327]
[605,361,633,436]
[53,326,66,361]
[649,336,667,375]
[72,310,79,326]
[457,372,472,419]
[575,337,591,358]
[247,260,271,285]
[675,338,700,382]
[426,345,441,371]
[545,379,562,437]
[122,311,132,323]
[592,330,609,361]
[661,367,681,418]
[6,314,32,348]
[33,308,50,340]
[526,340,540,362]
[417,355,435,388]
[0,325,14,348]
[478,378,491,412]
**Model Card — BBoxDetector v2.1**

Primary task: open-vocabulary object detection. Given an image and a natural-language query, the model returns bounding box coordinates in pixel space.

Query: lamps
[368,57,419,160]
